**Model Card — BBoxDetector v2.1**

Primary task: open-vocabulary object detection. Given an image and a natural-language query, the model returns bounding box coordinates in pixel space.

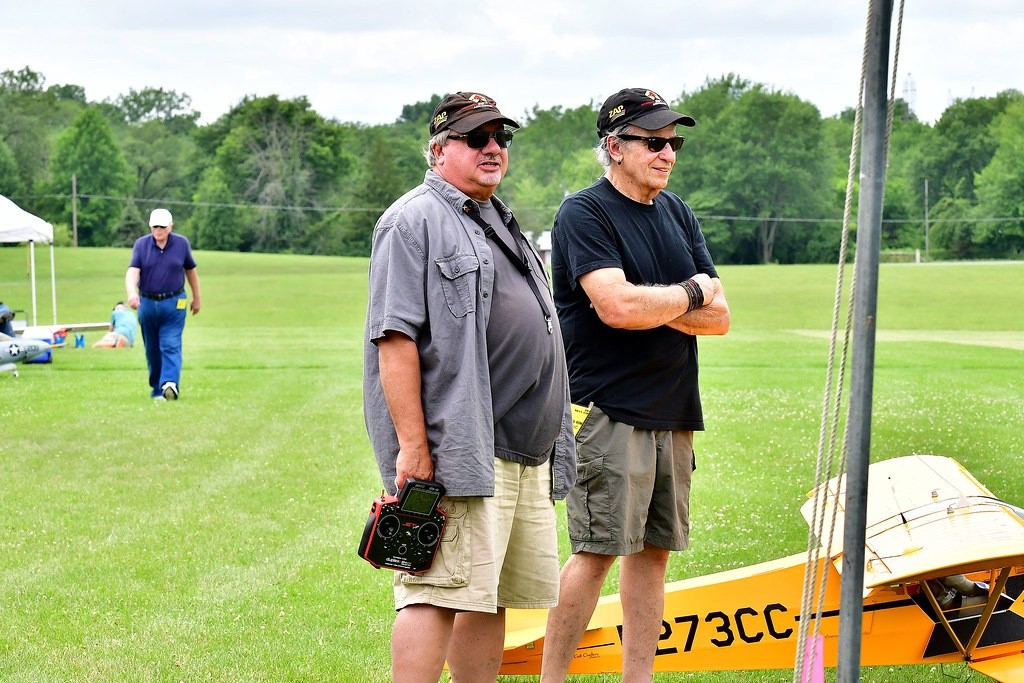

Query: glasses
[446,130,514,148]
[614,134,685,153]
[153,225,165,229]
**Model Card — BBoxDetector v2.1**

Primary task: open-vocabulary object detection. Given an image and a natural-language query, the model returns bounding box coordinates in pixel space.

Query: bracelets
[677,278,704,313]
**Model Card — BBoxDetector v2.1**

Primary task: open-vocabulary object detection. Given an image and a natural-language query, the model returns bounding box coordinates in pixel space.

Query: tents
[0,193,57,327]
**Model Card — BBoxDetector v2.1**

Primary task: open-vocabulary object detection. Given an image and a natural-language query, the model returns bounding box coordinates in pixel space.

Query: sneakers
[152,382,179,402]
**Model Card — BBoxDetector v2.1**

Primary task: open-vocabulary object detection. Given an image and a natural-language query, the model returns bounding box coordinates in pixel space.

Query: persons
[125,207,201,400]
[362,92,577,683]
[92,301,137,349]
[540,87,731,683]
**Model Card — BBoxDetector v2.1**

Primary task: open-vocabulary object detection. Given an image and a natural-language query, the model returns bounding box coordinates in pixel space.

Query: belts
[139,288,185,301]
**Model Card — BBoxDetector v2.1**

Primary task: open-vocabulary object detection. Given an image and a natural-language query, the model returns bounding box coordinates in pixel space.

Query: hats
[149,208,173,228]
[596,87,696,137]
[429,92,520,134]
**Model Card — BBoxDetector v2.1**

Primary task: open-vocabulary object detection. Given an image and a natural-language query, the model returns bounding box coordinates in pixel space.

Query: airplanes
[439,452,1024,683]
[0,331,69,376]
